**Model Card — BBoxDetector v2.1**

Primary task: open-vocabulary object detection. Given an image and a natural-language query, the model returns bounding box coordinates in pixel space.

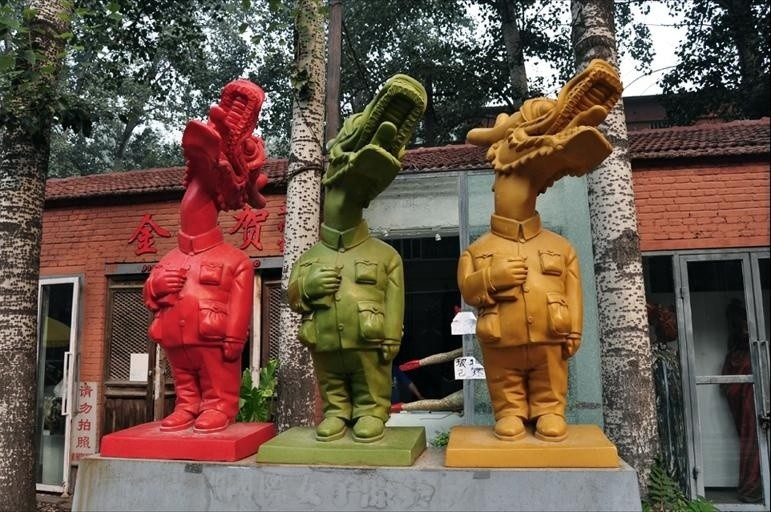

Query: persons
[719,297,764,503]
